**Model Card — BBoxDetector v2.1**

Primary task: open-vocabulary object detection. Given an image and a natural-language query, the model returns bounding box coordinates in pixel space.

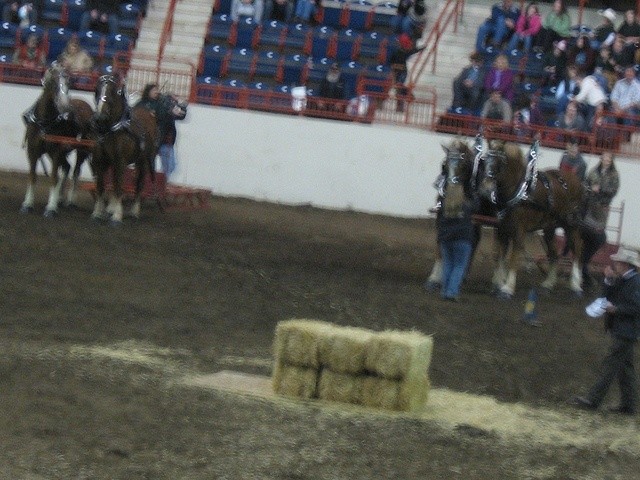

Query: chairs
[0,0,146,86]
[190,1,419,124]
[452,28,596,135]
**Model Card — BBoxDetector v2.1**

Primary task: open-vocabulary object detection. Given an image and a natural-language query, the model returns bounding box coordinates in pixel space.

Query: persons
[230,0,310,28]
[586,151,619,235]
[560,138,586,183]
[322,62,350,102]
[576,246,639,414]
[433,210,474,302]
[134,84,187,178]
[10,0,120,90]
[451,0,640,146]
[382,0,427,113]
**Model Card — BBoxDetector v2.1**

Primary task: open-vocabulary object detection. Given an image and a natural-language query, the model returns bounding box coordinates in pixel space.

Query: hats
[610,246,640,269]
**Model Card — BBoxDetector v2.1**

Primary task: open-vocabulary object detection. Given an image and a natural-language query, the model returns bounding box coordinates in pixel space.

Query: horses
[474,138,604,308]
[86,70,165,225]
[423,133,514,294]
[19,56,100,221]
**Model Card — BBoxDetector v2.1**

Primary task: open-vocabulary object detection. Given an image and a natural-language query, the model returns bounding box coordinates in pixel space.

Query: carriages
[429,132,626,301]
[20,61,212,224]
